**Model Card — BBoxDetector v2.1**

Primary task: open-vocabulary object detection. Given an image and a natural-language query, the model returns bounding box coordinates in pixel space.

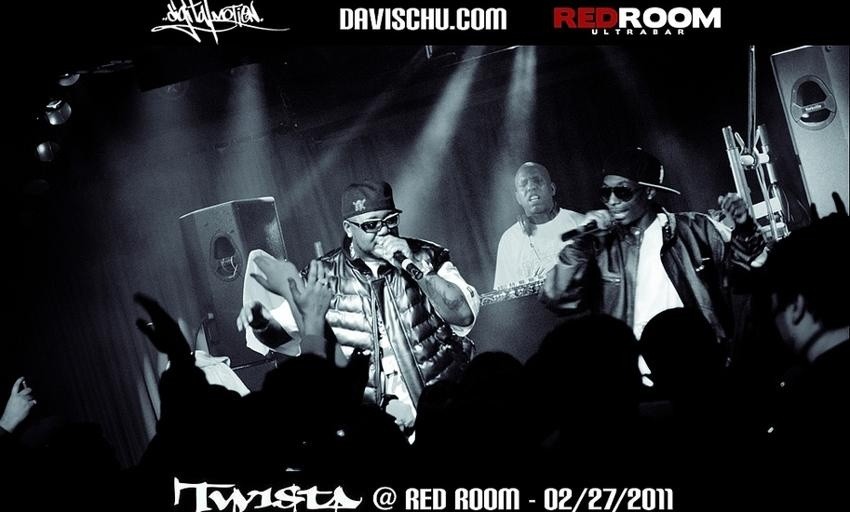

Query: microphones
[560,216,616,243]
[375,235,424,280]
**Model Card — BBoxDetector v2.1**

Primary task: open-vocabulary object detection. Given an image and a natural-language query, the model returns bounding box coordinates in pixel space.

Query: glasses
[345,212,405,232]
[598,186,641,204]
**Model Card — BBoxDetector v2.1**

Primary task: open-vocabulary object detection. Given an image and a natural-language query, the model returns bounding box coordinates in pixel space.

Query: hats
[607,148,681,193]
[339,181,406,219]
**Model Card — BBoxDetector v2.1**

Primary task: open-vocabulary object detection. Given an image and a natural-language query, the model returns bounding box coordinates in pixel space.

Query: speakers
[768,41,850,218]
[178,197,289,367]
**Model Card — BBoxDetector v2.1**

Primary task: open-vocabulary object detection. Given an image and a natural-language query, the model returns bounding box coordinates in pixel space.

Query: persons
[455,349,546,502]
[494,160,586,293]
[0,373,61,512]
[406,373,471,494]
[516,313,675,510]
[253,353,405,511]
[536,146,772,379]
[246,179,481,442]
[730,211,849,510]
[130,294,247,510]
[638,308,760,480]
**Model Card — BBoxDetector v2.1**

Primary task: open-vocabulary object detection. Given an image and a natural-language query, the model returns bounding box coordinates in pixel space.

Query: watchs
[410,259,433,281]
